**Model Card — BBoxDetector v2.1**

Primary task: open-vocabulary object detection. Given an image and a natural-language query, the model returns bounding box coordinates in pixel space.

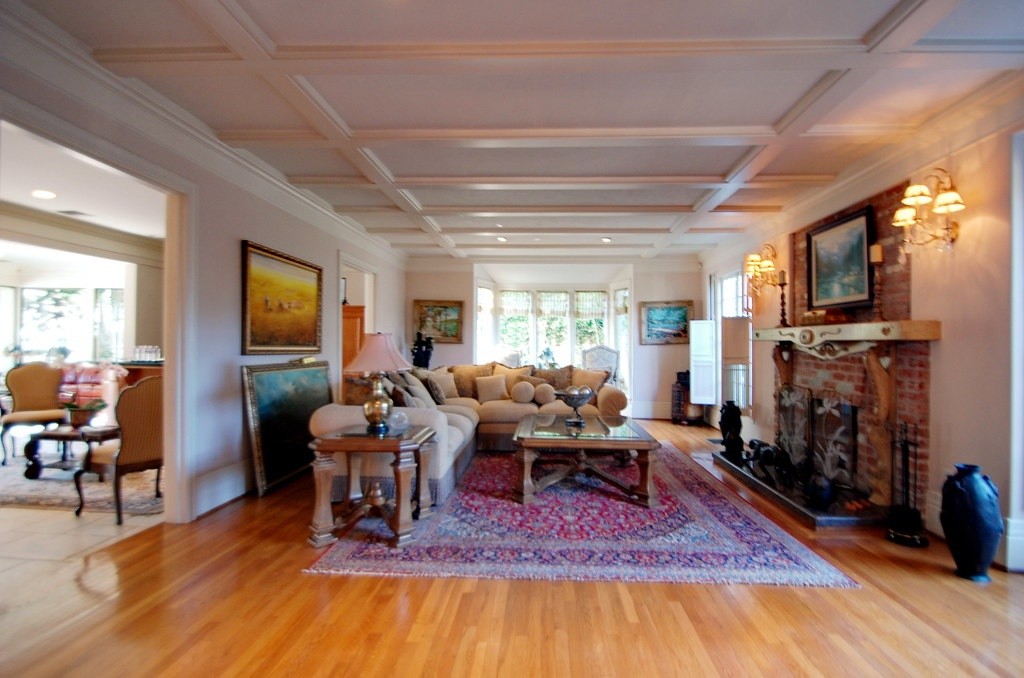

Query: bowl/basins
[535,414,556,426]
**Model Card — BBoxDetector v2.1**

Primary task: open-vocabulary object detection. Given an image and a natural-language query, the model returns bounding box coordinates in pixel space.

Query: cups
[130,345,161,360]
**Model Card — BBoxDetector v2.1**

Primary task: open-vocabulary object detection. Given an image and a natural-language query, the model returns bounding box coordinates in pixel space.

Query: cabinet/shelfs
[342,305,364,367]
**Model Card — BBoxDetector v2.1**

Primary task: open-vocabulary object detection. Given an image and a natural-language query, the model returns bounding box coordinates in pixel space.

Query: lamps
[891,164,962,255]
[742,244,777,297]
[342,329,415,435]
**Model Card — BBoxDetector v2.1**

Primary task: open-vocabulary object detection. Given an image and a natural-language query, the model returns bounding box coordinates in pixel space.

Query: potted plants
[61,396,107,426]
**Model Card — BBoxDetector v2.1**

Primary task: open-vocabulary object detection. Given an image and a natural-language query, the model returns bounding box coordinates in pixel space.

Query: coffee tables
[513,415,662,505]
[25,424,121,482]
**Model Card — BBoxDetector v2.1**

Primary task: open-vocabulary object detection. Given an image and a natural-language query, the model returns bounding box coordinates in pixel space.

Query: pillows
[382,360,612,418]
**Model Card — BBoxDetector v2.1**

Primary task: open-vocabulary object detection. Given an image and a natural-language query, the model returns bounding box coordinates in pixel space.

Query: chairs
[0,362,67,468]
[580,346,623,388]
[74,375,165,524]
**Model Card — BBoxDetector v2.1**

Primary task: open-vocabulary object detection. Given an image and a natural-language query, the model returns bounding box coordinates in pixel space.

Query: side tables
[305,424,433,547]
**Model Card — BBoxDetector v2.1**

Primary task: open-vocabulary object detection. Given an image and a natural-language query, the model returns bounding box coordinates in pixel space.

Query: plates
[113,359,165,365]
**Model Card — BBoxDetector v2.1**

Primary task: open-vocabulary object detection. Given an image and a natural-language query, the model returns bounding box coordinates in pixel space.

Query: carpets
[304,436,859,591]
[0,431,166,514]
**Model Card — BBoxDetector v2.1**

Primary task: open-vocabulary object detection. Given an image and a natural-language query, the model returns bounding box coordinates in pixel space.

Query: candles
[870,242,883,262]
[778,267,786,285]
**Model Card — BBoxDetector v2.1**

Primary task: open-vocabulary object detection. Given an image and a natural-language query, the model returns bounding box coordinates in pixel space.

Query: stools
[333,361,628,505]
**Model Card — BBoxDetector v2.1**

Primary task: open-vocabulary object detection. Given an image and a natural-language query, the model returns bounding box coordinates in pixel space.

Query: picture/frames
[242,357,329,492]
[241,239,324,356]
[805,204,878,312]
[639,298,695,347]
[412,299,466,344]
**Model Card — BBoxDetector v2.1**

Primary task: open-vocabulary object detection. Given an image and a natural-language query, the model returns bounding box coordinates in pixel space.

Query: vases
[718,399,744,444]
[940,462,1005,583]
